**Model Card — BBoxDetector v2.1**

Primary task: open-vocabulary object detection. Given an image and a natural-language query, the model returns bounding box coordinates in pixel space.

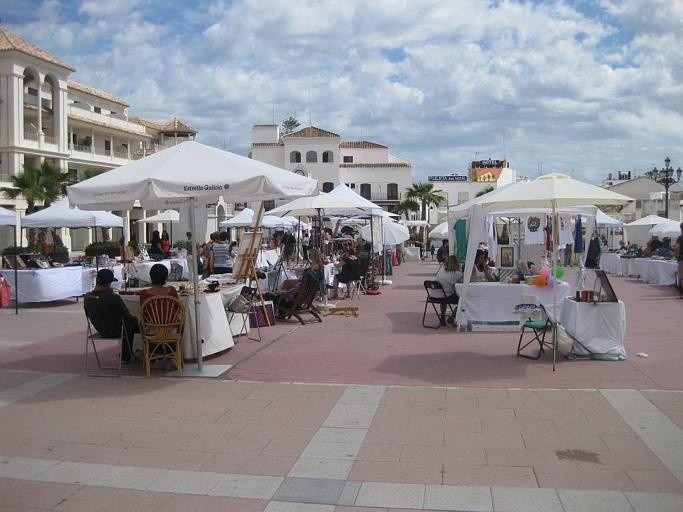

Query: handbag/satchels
[228,293,251,312]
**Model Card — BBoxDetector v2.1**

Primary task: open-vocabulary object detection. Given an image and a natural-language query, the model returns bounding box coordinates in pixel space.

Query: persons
[34,221,402,364]
[429,223,683,326]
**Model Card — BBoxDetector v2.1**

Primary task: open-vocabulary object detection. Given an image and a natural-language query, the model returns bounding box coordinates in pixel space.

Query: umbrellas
[136,210,180,248]
[472,172,636,371]
[66,140,319,370]
[626,215,667,228]
[264,190,328,249]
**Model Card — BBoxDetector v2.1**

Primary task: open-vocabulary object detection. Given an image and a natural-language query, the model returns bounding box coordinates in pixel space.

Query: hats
[96,269,118,282]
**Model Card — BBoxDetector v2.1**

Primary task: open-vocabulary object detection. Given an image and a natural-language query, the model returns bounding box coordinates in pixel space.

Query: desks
[454,251,683,362]
[0,242,356,362]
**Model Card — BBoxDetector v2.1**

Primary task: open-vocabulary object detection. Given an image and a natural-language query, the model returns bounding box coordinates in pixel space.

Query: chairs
[86,294,186,378]
[433,253,443,276]
[422,281,456,330]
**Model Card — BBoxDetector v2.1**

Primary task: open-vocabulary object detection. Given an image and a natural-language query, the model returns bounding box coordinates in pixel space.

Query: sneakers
[440,317,447,326]
[119,355,138,364]
[447,318,456,327]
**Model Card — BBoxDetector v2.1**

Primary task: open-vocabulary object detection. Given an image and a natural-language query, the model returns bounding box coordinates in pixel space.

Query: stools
[342,276,366,301]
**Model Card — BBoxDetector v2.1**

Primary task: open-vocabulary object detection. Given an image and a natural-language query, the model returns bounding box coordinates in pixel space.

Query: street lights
[651,157,681,217]
